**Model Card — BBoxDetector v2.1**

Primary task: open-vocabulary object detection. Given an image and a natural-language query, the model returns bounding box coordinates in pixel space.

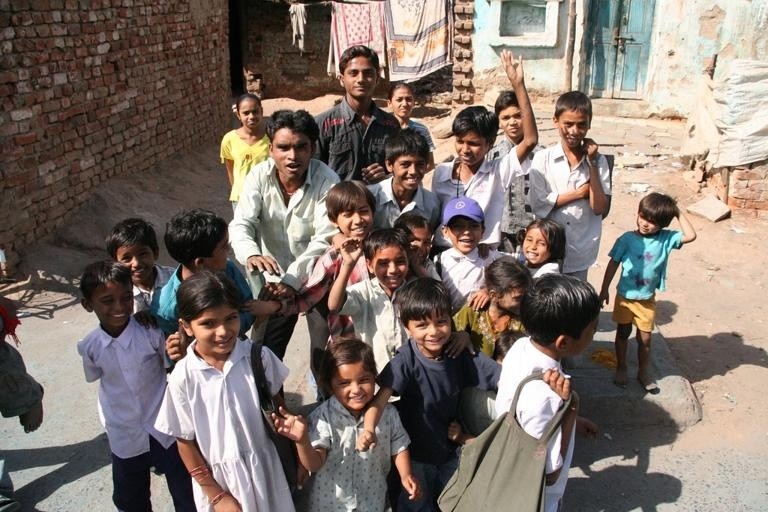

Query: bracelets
[190,465,208,476]
[210,491,226,504]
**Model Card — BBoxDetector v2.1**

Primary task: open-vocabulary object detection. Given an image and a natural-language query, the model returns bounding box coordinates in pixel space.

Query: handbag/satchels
[260,393,297,496]
[436,409,548,512]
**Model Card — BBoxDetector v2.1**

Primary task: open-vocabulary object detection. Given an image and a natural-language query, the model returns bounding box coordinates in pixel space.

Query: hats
[443,197,484,225]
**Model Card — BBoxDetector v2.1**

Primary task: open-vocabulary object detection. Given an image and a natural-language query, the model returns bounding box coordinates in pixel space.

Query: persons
[272,337,422,512]
[1,296,46,511]
[156,46,612,435]
[490,274,599,509]
[104,217,178,475]
[598,191,698,394]
[75,259,196,512]
[154,271,290,511]
[356,276,502,511]
